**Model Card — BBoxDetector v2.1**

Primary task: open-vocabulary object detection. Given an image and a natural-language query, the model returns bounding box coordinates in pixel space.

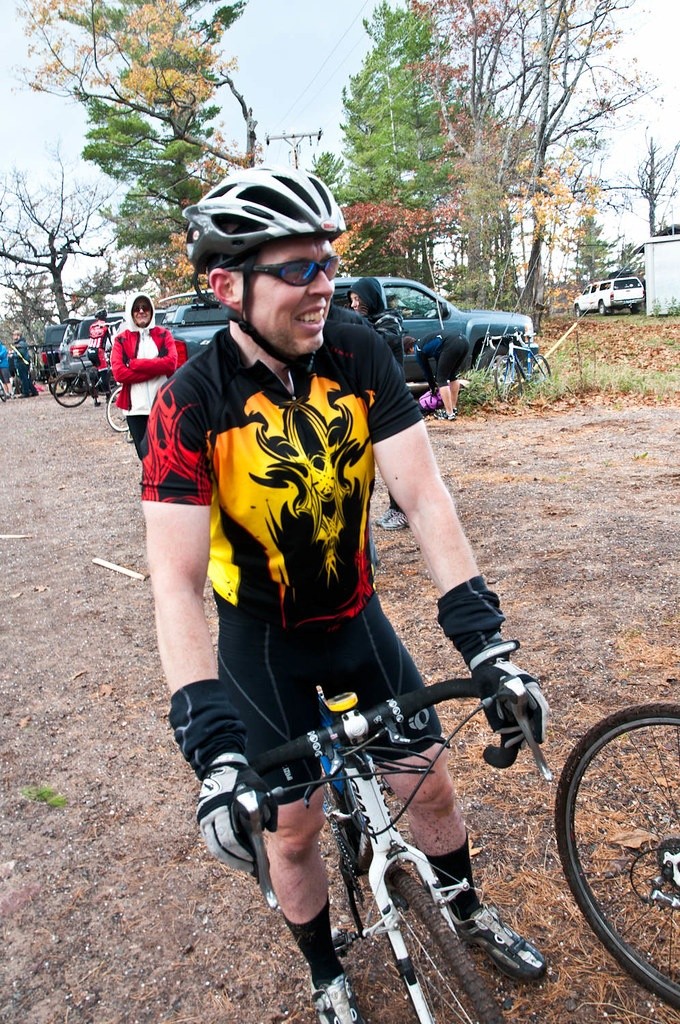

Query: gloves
[434,575,547,770]
[158,348,168,357]
[168,678,277,877]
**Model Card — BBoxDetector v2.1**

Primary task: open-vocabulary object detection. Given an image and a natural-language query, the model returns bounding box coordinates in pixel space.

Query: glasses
[224,253,342,286]
[133,305,152,312]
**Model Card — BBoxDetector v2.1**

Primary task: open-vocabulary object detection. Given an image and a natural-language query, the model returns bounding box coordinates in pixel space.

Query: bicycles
[102,382,137,444]
[252,675,552,1024]
[52,345,118,408]
[485,330,551,403]
[552,703,680,1008]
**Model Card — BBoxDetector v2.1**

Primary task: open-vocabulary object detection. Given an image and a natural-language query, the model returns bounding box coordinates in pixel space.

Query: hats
[403,336,415,352]
[12,330,22,336]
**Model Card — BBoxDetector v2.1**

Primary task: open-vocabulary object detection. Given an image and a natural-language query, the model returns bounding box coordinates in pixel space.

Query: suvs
[56,309,170,378]
[571,277,646,316]
[37,324,67,382]
[159,276,537,388]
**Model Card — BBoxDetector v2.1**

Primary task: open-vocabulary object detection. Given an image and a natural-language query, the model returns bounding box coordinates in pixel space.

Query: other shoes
[6,393,16,399]
[30,392,38,396]
[106,394,117,403]
[18,393,28,398]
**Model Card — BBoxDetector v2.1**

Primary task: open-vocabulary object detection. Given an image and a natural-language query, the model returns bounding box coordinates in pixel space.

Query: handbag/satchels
[419,382,451,414]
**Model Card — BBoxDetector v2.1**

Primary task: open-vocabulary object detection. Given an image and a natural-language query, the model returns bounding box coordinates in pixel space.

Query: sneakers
[439,408,458,420]
[308,973,364,1024]
[375,509,409,531]
[446,906,546,981]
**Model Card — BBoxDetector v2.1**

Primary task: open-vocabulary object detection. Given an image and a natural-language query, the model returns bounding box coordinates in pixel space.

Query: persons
[0,339,16,398]
[12,330,39,398]
[86,309,118,405]
[404,329,470,419]
[346,278,409,531]
[109,292,179,457]
[138,168,549,1024]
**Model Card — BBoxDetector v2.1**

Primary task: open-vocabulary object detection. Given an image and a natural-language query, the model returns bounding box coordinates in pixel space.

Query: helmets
[182,165,347,274]
[94,308,107,317]
